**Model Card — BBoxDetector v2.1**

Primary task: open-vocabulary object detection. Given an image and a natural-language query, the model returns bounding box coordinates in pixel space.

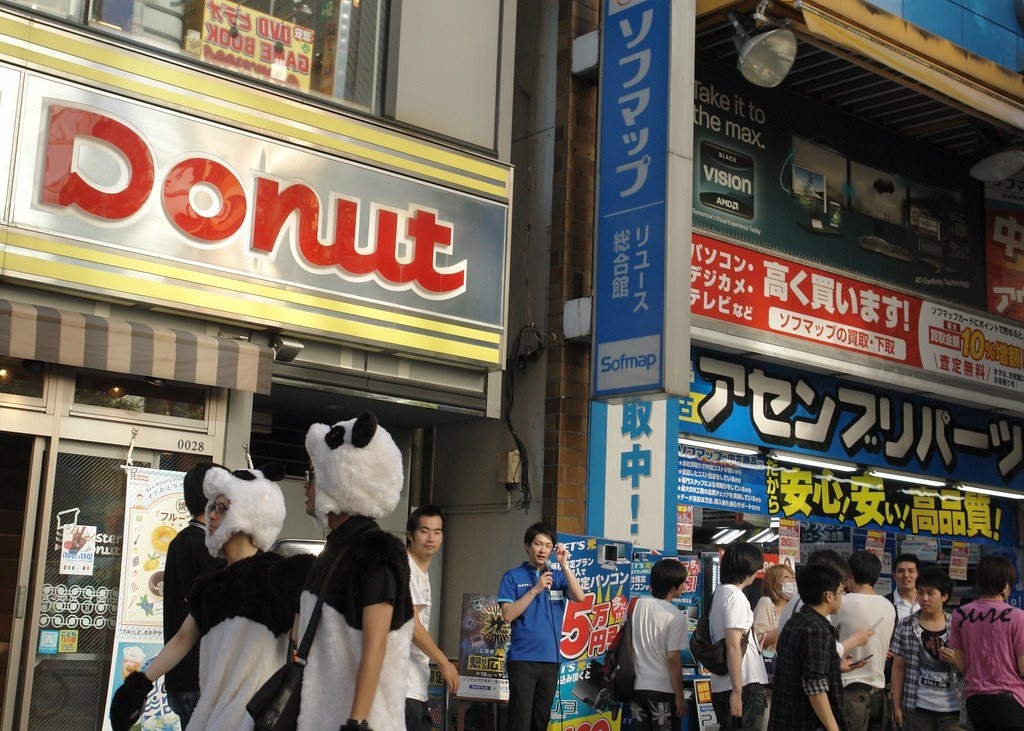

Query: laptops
[614,543,631,564]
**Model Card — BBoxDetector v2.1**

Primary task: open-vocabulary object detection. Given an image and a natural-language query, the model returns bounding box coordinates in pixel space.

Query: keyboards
[601,564,616,570]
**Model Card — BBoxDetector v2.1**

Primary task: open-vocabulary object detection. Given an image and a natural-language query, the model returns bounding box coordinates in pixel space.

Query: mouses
[615,567,618,571]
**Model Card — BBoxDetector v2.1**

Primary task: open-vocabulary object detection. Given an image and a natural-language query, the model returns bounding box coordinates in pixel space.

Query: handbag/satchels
[246,662,305,731]
[603,596,639,702]
[689,615,748,676]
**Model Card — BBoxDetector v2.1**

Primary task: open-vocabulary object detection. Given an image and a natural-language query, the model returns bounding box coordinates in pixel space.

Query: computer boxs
[597,545,609,564]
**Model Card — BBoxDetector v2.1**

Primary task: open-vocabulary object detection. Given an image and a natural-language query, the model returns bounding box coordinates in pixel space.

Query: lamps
[283,6,312,20]
[0,363,14,386]
[108,382,129,399]
[964,126,1023,183]
[269,336,305,365]
[145,378,170,389]
[727,1,801,88]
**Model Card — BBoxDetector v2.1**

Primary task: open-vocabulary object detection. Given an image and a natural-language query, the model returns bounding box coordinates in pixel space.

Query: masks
[777,580,798,600]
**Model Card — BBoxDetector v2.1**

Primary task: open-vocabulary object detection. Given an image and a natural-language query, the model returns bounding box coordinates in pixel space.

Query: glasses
[209,503,228,514]
[305,469,315,483]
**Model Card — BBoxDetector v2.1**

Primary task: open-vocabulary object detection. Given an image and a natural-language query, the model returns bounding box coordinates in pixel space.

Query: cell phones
[849,654,874,666]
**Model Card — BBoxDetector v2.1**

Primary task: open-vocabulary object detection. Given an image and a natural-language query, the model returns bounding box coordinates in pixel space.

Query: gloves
[110,671,154,731]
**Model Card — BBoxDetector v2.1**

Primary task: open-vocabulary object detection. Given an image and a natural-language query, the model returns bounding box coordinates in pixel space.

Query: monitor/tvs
[791,164,826,214]
[604,544,618,566]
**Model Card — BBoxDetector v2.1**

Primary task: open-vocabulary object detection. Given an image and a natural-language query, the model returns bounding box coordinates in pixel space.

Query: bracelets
[561,566,570,570]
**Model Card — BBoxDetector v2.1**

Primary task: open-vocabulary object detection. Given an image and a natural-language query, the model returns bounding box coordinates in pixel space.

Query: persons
[163,462,232,731]
[753,548,896,731]
[293,410,415,731]
[405,504,460,731]
[706,542,769,731]
[890,566,961,731]
[110,462,301,731]
[496,523,585,731]
[948,556,1024,731]
[620,559,690,731]
[883,553,924,626]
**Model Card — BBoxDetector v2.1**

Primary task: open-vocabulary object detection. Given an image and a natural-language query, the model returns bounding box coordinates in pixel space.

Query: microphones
[544,559,551,591]
